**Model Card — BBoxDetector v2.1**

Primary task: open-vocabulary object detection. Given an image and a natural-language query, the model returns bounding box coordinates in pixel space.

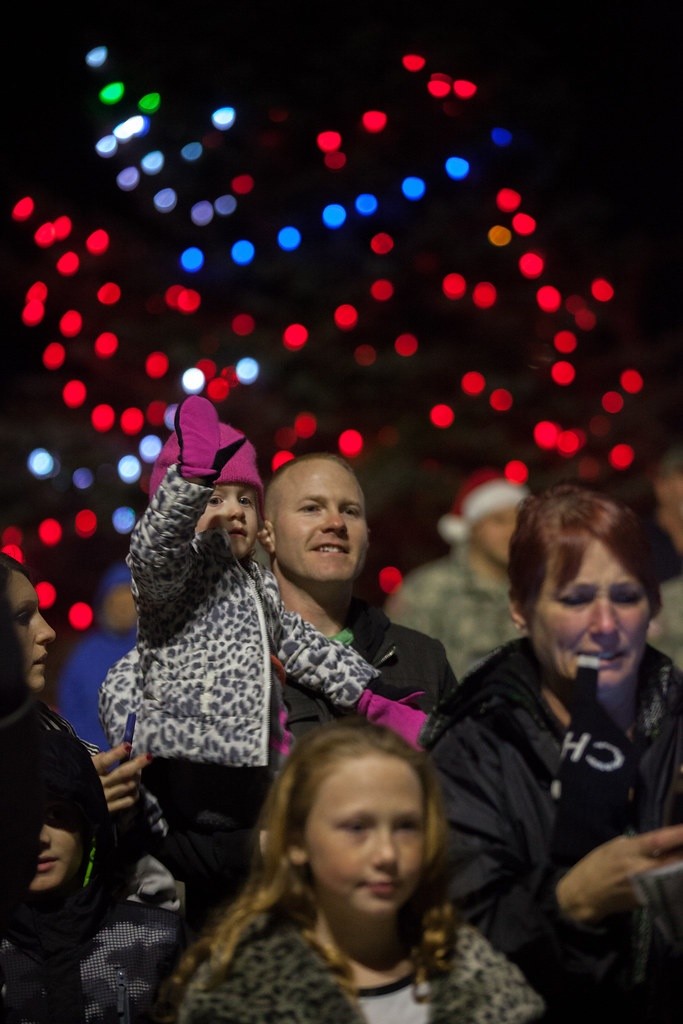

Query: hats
[437,470,533,541]
[149,423,265,520]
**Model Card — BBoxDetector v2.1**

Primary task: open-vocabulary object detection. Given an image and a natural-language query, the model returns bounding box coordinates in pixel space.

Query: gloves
[174,397,245,480]
[359,678,431,749]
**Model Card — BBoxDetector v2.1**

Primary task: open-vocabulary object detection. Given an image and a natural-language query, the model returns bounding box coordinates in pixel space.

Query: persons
[62,566,141,764]
[259,453,455,746]
[99,394,430,924]
[383,470,533,686]
[420,486,683,1023]
[0,556,151,814]
[648,449,682,667]
[165,722,544,1024]
[0,732,193,1023]
